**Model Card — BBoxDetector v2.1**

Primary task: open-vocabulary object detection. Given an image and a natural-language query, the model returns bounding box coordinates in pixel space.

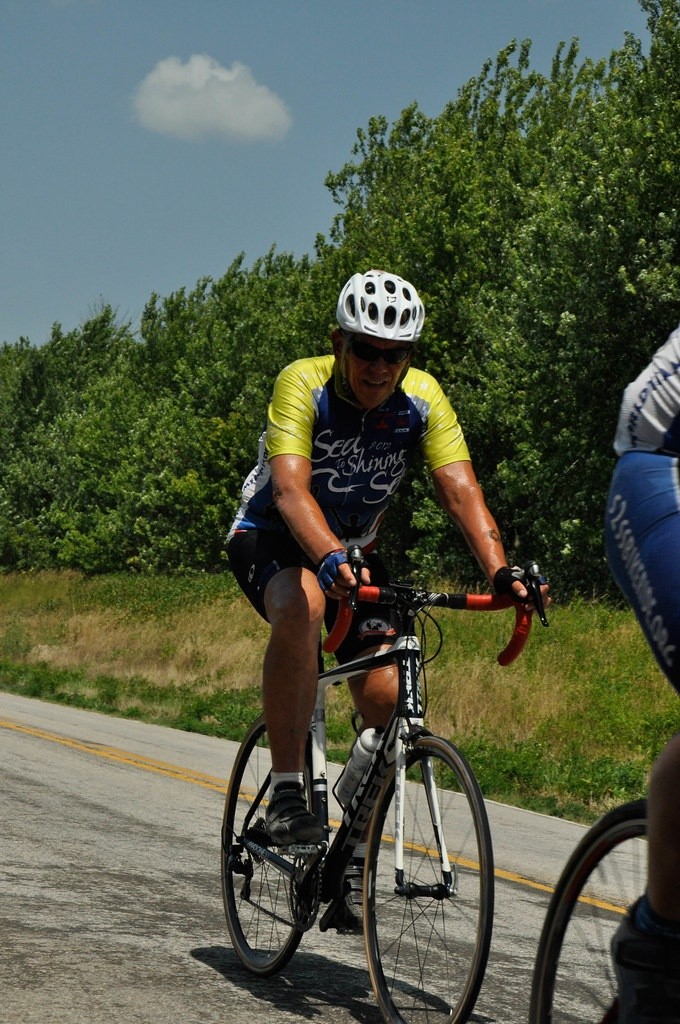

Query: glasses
[339,328,412,364]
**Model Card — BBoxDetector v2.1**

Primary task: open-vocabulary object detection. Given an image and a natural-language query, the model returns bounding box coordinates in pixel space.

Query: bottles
[337,725,385,807]
[238,519,258,553]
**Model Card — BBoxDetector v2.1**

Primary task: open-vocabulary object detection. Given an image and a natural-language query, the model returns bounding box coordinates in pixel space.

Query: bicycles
[219,544,553,1024]
[523,797,680,1024]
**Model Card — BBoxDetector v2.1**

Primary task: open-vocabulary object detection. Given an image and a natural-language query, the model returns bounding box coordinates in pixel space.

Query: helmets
[336,269,425,341]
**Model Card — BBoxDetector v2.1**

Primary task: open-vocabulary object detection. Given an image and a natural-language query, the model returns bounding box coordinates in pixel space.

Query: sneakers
[332,900,379,930]
[610,895,680,1024]
[261,789,326,844]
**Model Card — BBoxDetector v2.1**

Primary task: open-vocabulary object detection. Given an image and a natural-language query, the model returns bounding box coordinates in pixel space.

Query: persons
[225,268,552,933]
[600,324,680,1024]
